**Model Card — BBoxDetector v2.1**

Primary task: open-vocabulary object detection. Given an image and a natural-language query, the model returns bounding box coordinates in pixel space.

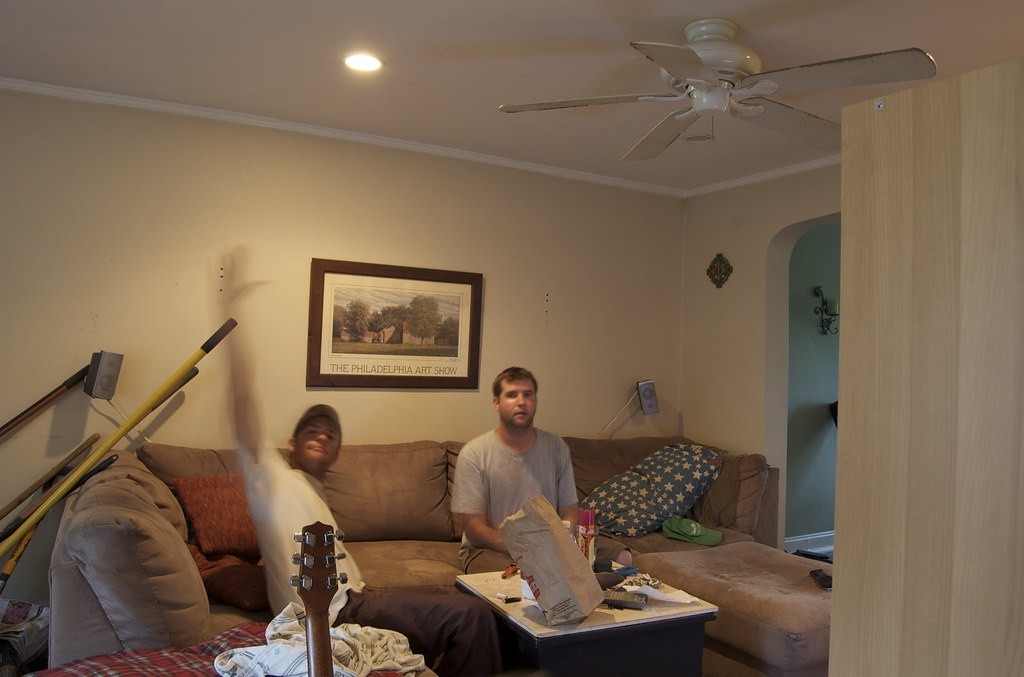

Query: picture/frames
[305,257,483,389]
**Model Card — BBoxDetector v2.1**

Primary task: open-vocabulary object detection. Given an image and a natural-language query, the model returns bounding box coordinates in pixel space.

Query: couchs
[43,436,832,677]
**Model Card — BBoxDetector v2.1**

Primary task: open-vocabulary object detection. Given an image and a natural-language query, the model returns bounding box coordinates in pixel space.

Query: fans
[497,20,938,164]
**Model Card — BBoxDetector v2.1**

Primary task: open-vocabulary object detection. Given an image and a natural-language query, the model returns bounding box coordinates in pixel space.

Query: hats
[292,403,342,449]
[662,518,723,546]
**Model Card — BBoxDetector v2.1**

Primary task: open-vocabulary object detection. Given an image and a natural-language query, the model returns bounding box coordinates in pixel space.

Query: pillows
[171,471,259,556]
[578,443,722,538]
[185,543,270,612]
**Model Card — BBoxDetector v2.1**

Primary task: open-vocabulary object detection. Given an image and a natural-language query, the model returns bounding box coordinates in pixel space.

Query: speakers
[636,379,660,416]
[84,350,124,400]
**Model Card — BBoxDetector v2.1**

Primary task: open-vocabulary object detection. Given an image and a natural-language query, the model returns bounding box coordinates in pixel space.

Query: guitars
[291,519,349,677]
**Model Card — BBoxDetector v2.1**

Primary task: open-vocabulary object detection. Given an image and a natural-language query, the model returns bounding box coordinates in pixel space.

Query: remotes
[614,566,637,576]
[602,590,648,610]
[810,568,832,588]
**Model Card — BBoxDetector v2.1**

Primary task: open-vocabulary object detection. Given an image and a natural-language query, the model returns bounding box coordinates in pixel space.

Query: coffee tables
[456,559,719,677]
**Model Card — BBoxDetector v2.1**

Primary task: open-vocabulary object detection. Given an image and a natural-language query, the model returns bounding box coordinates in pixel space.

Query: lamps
[814,287,840,335]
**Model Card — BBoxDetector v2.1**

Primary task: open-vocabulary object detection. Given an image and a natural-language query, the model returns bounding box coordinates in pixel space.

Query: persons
[451,366,633,576]
[214,248,499,676]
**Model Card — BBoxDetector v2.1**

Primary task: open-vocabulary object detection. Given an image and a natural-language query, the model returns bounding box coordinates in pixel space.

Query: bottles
[520,565,536,600]
[557,518,577,547]
